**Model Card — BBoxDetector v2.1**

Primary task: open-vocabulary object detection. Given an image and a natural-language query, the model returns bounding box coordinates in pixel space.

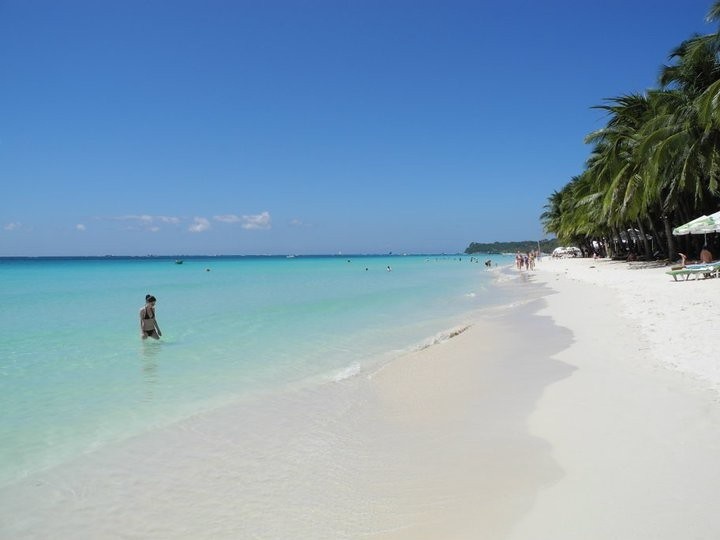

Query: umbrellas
[673,211,720,246]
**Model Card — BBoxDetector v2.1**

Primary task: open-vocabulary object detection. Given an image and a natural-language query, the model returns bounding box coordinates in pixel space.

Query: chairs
[666,262,720,281]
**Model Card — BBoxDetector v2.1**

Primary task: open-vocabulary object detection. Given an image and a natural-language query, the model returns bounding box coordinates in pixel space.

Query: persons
[139,294,162,340]
[459,258,462,261]
[516,249,543,271]
[678,246,714,266]
[485,260,492,268]
